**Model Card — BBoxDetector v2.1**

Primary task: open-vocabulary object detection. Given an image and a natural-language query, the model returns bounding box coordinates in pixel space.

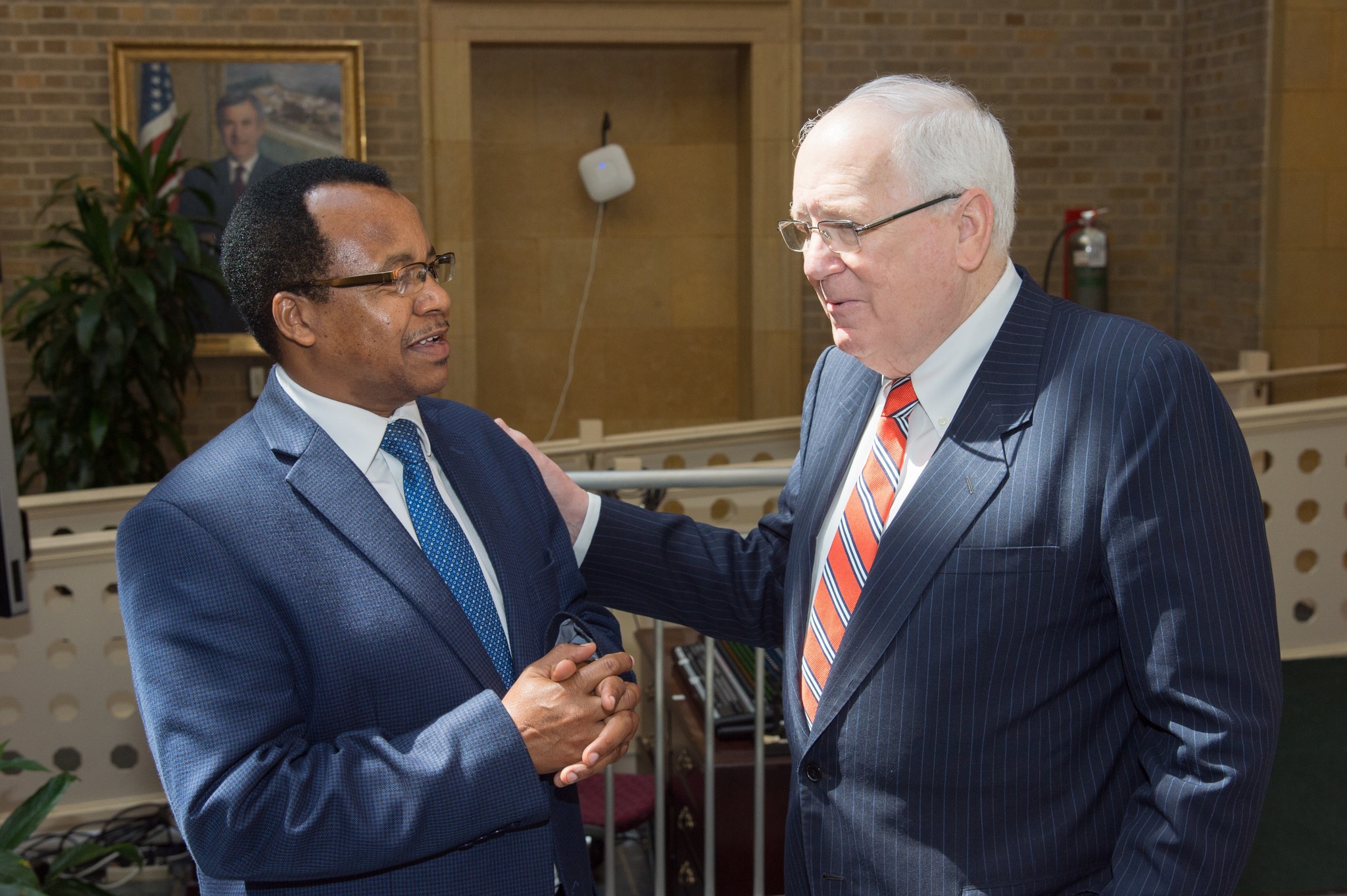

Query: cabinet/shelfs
[634,624,792,896]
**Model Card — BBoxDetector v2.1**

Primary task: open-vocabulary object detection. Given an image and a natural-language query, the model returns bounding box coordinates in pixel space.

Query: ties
[800,376,920,736]
[379,418,517,692]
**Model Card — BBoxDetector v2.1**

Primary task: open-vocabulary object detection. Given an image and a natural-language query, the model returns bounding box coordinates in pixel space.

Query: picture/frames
[107,38,366,359]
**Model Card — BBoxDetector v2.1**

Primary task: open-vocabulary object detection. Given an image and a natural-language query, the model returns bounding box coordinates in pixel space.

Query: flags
[136,59,182,244]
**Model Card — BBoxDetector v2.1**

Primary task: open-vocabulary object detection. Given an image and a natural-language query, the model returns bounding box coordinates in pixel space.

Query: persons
[176,88,285,331]
[117,155,640,896]
[480,75,1284,896]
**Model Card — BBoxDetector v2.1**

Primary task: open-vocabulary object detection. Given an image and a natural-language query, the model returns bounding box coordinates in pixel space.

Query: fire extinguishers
[1040,207,1113,315]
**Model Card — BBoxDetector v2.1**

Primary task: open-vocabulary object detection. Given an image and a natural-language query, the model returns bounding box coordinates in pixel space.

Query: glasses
[776,193,964,253]
[264,252,455,313]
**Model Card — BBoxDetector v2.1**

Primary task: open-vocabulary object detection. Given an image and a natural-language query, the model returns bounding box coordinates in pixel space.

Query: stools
[576,773,656,896]
